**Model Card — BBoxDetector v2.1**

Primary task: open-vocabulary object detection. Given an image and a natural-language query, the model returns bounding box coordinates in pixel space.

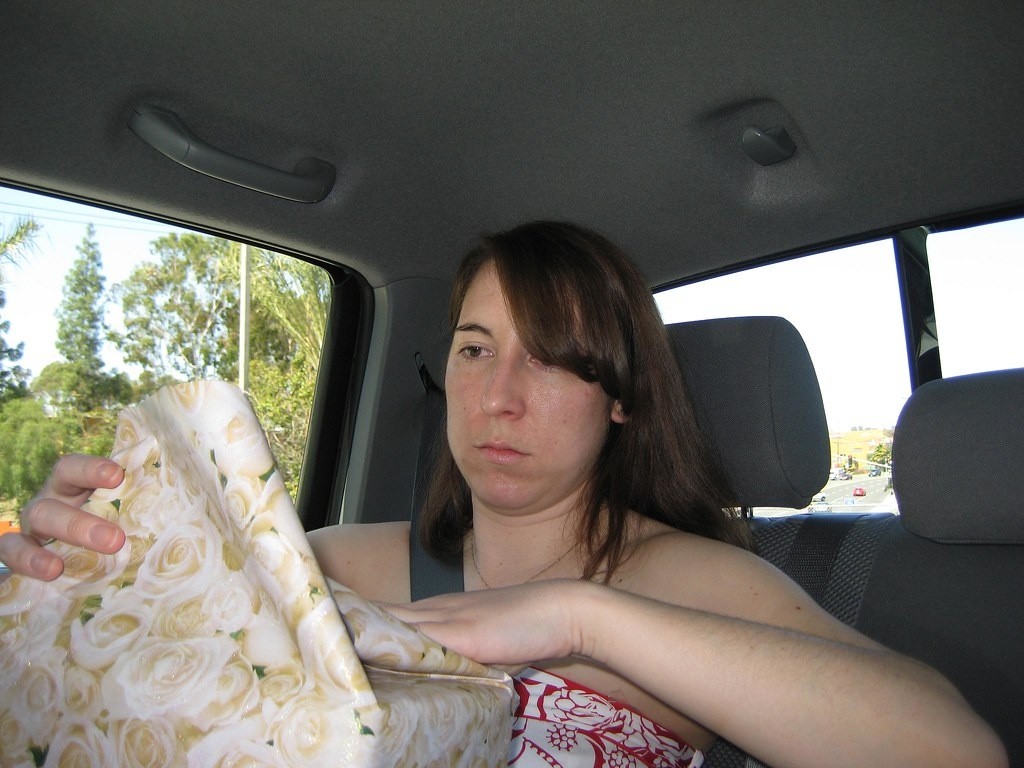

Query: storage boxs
[0,379,516,768]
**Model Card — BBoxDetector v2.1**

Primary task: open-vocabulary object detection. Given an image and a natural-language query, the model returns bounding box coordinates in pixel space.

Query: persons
[1,218,1010,768]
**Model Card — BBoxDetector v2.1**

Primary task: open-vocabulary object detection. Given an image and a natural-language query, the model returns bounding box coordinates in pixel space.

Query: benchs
[665,316,1024,768]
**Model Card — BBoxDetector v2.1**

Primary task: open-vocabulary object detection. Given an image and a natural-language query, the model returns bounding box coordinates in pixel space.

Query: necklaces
[471,529,579,588]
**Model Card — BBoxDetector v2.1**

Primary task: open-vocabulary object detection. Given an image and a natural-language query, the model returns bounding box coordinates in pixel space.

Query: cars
[852,487,867,496]
[829,467,853,481]
[811,492,827,502]
[869,469,881,477]
